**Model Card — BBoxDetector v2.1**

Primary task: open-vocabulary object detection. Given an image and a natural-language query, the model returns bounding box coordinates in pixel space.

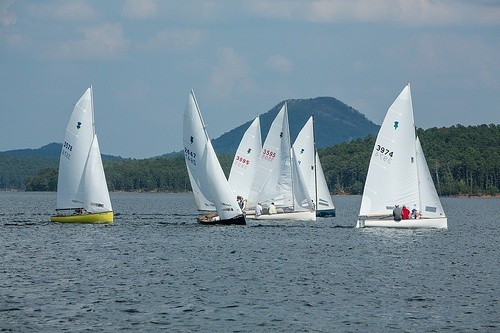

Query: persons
[408,209,417,219]
[267,202,277,214]
[255,203,262,216]
[401,206,410,220]
[393,204,404,221]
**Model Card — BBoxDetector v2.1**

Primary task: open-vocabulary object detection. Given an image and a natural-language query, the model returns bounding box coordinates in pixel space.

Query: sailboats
[355,83,449,229]
[227,115,262,205]
[49,84,114,224]
[181,88,248,225]
[243,101,318,222]
[290,113,337,217]
